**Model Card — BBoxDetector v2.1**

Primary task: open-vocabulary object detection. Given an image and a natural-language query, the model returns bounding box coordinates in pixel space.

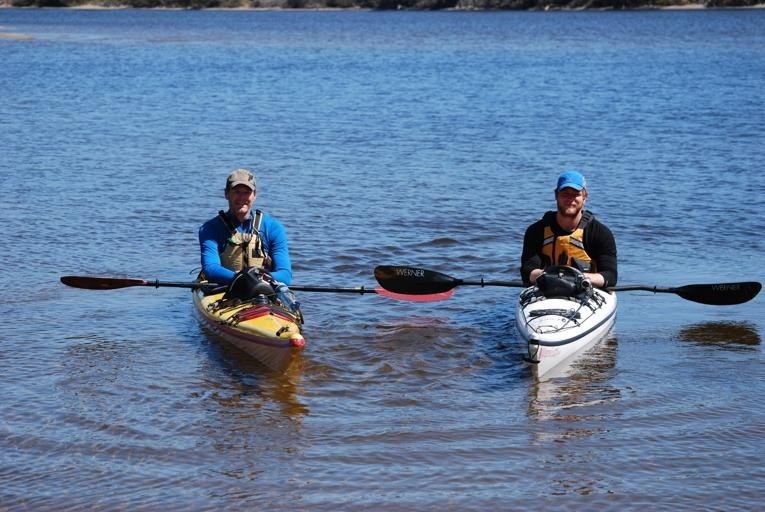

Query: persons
[519,169,618,302]
[199,169,293,293]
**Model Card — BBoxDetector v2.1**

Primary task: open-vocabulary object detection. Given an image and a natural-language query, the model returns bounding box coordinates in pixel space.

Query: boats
[515,285,617,383]
[191,269,305,373]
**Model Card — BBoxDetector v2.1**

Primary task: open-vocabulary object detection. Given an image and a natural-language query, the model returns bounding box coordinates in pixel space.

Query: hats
[226,169,255,190]
[557,171,586,191]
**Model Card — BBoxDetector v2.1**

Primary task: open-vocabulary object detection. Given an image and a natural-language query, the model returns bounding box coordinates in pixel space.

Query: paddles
[59,272,455,303]
[375,263,762,308]
